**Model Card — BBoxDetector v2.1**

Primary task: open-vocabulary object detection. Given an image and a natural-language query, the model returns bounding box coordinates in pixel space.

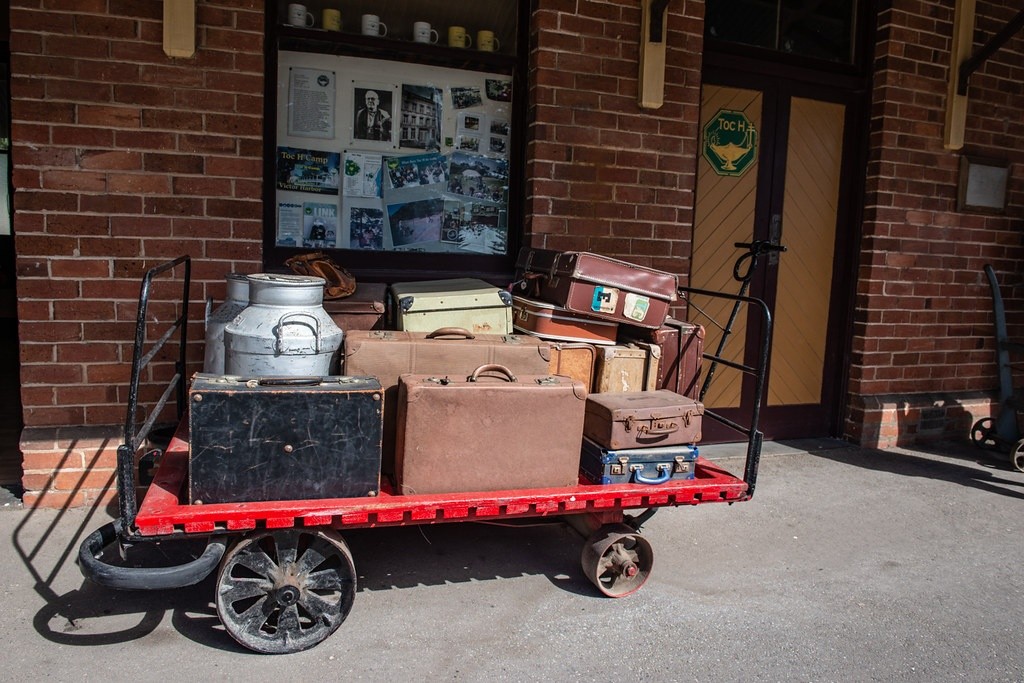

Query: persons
[309,218,327,241]
[355,90,391,141]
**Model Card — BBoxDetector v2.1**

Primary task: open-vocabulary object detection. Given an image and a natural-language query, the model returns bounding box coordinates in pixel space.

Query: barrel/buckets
[201,271,344,379]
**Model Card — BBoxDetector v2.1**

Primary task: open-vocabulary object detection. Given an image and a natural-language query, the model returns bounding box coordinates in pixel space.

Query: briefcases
[186,246,706,504]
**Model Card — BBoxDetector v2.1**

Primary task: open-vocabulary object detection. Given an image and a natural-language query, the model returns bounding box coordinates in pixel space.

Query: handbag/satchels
[284,251,358,299]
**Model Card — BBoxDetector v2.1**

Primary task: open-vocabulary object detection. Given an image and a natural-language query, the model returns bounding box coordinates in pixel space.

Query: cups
[287,3,314,28]
[362,14,386,37]
[414,21,438,44]
[477,30,500,52]
[323,9,340,32]
[450,26,472,48]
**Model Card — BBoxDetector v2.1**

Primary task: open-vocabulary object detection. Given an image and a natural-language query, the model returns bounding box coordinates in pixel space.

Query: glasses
[366,98,378,100]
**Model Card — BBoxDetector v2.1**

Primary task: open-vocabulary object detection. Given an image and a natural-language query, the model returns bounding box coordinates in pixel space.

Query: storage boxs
[390,278,514,332]
[323,283,389,358]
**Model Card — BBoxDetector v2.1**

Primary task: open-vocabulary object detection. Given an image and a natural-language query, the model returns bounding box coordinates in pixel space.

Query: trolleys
[971,264,1024,473]
[73,253,773,656]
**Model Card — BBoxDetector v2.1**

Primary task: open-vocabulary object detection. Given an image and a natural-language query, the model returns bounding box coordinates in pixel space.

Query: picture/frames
[956,154,1014,216]
